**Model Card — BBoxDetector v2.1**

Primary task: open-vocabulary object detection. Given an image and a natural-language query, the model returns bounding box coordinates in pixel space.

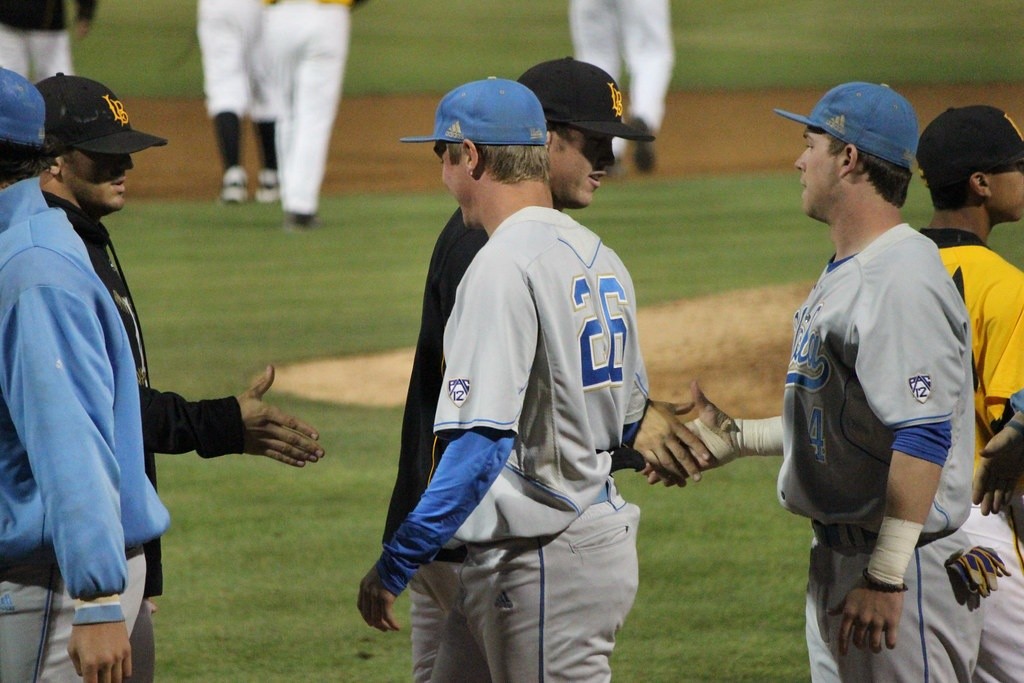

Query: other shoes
[255,168,280,204]
[222,166,249,204]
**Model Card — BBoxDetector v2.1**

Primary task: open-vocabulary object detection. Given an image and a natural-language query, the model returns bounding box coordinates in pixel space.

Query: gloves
[948,546,1013,599]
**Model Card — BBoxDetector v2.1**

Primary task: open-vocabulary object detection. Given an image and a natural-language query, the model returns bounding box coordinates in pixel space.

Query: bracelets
[863,568,908,593]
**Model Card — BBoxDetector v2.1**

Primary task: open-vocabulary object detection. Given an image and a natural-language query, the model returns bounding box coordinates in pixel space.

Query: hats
[775,81,920,172]
[0,66,47,145]
[400,76,547,146]
[518,54,656,144]
[35,71,169,155]
[916,105,1024,188]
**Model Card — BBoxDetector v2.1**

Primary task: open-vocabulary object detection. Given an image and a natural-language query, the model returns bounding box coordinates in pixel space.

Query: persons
[384,56,710,683]
[971,389,1024,517]
[36,73,327,683]
[0,0,102,87]
[195,0,365,230]
[566,0,676,179]
[2,68,169,683]
[911,104,1024,683]
[643,78,981,683]
[355,77,642,683]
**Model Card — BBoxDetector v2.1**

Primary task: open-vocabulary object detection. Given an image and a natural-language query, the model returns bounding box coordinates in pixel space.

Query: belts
[807,517,936,553]
